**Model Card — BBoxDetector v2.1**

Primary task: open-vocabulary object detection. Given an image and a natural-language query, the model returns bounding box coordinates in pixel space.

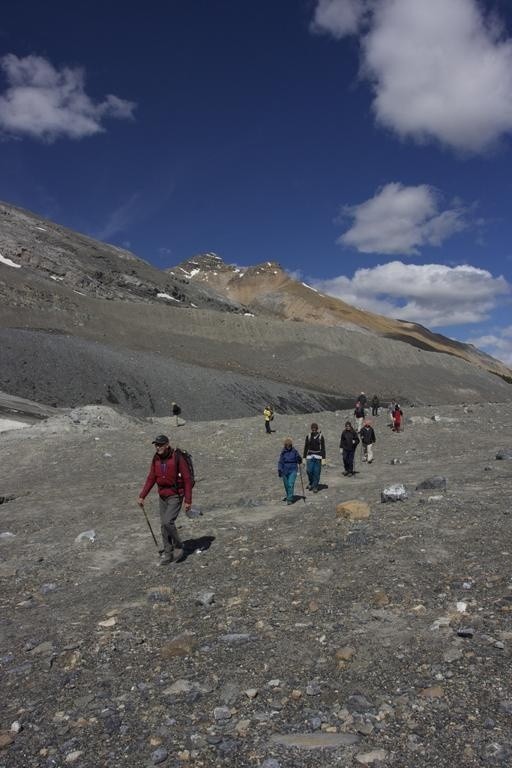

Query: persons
[303,422,326,493]
[137,435,193,566]
[353,391,368,434]
[263,405,273,434]
[278,439,305,506]
[355,420,376,464]
[171,402,179,427]
[339,421,360,477]
[370,395,380,417]
[387,398,404,433]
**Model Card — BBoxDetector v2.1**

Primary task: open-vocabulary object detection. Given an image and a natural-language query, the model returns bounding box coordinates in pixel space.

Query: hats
[152,402,184,444]
[284,419,374,447]
[352,390,401,422]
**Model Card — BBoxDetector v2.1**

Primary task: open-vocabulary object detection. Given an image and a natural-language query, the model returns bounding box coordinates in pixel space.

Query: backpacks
[270,411,274,422]
[182,449,196,489]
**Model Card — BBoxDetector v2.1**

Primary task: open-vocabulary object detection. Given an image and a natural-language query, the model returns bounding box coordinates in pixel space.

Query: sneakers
[162,548,184,563]
[281,456,377,505]
